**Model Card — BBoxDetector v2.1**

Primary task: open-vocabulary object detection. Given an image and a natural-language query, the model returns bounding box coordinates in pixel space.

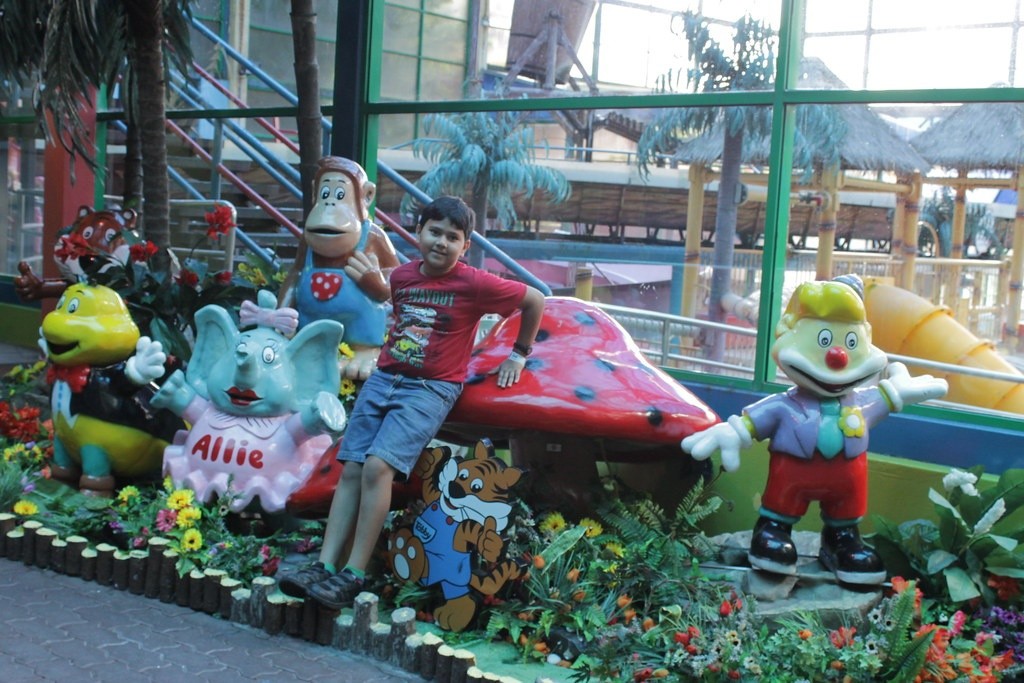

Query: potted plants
[939,185,1004,258]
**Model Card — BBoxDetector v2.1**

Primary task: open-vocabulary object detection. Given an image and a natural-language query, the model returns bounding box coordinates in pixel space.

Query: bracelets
[507,350,528,365]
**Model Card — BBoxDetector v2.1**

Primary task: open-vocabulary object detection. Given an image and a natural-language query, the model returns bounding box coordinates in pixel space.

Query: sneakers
[279,561,333,599]
[304,568,374,608]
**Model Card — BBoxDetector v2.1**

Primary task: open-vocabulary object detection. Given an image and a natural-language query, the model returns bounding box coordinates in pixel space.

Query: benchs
[238,163,1016,259]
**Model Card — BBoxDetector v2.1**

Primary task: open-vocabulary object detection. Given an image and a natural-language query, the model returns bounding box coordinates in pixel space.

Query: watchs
[513,341,533,354]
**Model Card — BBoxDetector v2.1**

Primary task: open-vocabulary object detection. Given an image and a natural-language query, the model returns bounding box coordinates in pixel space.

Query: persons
[279,196,544,608]
